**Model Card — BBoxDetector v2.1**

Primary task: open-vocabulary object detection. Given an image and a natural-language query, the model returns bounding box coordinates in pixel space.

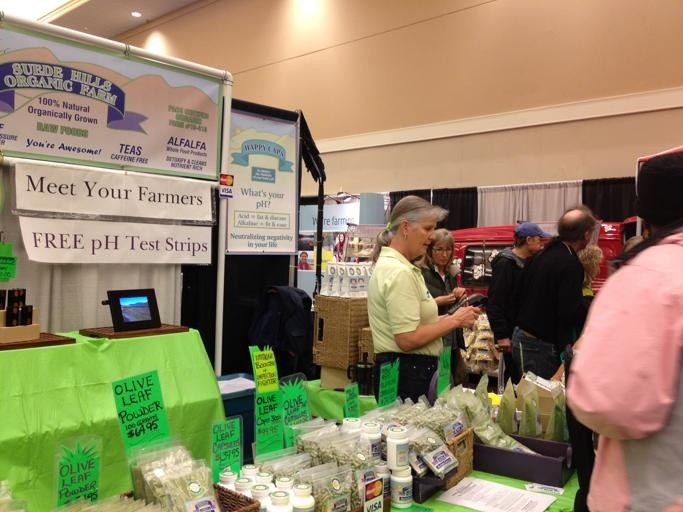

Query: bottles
[336,416,414,510]
[217,465,315,512]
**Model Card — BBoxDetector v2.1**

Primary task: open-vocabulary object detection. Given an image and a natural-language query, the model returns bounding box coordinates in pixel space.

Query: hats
[636,151,683,223]
[514,223,551,238]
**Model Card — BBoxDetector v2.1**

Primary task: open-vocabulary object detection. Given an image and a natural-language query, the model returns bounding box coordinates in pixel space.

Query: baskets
[213,481,260,512]
[442,426,475,489]
[312,294,374,371]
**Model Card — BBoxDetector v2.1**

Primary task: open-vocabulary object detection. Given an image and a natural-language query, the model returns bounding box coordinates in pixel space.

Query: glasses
[432,248,453,253]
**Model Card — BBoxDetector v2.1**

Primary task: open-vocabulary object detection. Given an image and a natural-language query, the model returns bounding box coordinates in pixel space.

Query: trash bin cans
[213,372,255,464]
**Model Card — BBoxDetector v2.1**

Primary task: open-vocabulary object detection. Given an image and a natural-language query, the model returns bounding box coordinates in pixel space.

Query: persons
[575,242,601,296]
[298,251,313,270]
[485,222,553,394]
[418,227,466,386]
[564,148,681,512]
[510,203,601,384]
[331,231,345,262]
[365,193,484,404]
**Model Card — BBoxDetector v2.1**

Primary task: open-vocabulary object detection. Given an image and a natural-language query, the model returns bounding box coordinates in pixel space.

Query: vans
[443,220,625,318]
